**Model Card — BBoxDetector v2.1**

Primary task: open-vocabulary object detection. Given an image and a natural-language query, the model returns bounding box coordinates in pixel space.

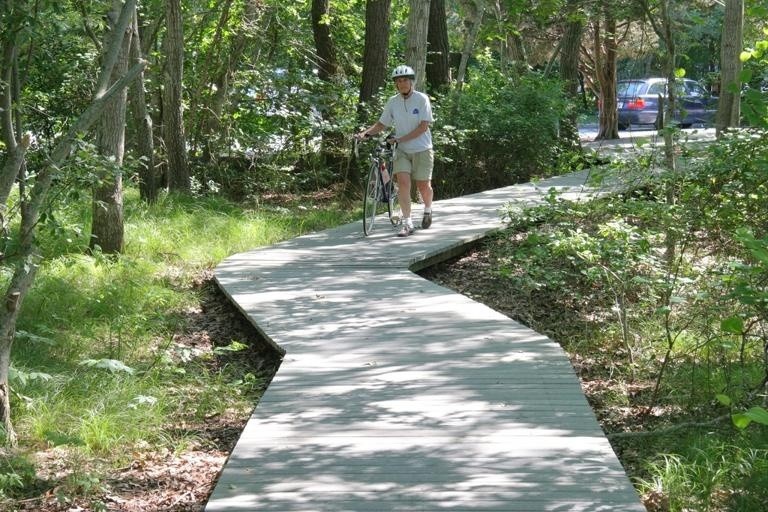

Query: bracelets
[396,136,401,143]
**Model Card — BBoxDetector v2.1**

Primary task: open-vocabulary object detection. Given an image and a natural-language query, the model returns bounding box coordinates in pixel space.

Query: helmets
[392,65,415,81]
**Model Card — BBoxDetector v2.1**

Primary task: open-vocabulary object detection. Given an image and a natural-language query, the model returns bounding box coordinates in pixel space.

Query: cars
[596,75,719,128]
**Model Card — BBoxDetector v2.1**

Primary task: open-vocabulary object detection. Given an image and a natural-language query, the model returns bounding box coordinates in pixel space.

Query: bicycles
[348,128,403,236]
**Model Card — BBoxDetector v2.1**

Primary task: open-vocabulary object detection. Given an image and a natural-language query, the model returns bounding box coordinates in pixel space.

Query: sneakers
[422,209,432,229]
[398,224,415,237]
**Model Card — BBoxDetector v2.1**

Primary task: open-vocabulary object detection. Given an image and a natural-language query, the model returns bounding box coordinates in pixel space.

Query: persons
[358,65,435,237]
